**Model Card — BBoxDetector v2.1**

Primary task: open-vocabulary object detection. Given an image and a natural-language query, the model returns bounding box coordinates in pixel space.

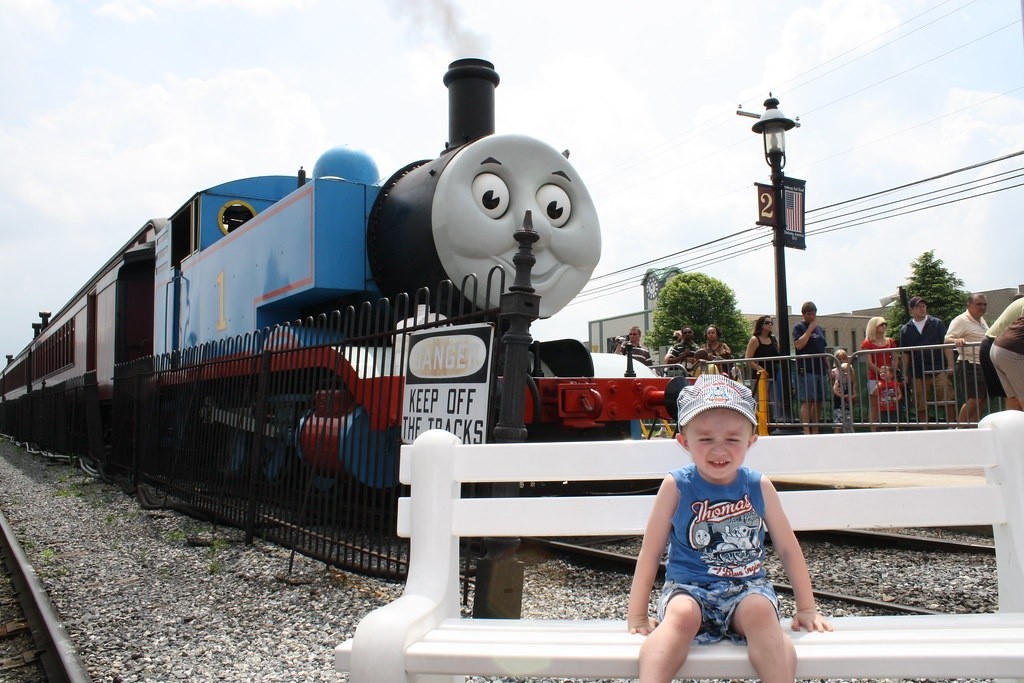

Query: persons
[900,296,956,430]
[979,297,1024,398]
[831,349,857,433]
[745,315,780,422]
[792,302,834,434]
[732,363,741,378]
[613,326,653,366]
[989,316,1024,412]
[944,293,990,429]
[860,315,902,432]
[664,325,734,377]
[627,373,833,683]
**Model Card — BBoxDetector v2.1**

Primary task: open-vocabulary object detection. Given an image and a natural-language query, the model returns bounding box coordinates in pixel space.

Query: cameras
[616,337,626,342]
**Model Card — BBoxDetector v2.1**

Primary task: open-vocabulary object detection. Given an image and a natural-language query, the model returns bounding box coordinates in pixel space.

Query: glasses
[684,331,693,335]
[629,332,640,336]
[880,322,888,327]
[761,321,774,326]
[969,303,987,306]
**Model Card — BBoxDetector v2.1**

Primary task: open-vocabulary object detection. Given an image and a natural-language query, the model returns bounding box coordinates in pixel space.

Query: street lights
[750,92,795,420]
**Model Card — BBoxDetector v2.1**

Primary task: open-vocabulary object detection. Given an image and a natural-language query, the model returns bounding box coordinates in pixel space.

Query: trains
[0,59,697,507]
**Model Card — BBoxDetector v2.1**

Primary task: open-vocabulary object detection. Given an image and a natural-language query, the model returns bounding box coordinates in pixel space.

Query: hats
[910,297,925,308]
[677,373,758,434]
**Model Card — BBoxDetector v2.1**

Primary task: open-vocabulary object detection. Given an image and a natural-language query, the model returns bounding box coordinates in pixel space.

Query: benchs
[333,410,1024,683]
